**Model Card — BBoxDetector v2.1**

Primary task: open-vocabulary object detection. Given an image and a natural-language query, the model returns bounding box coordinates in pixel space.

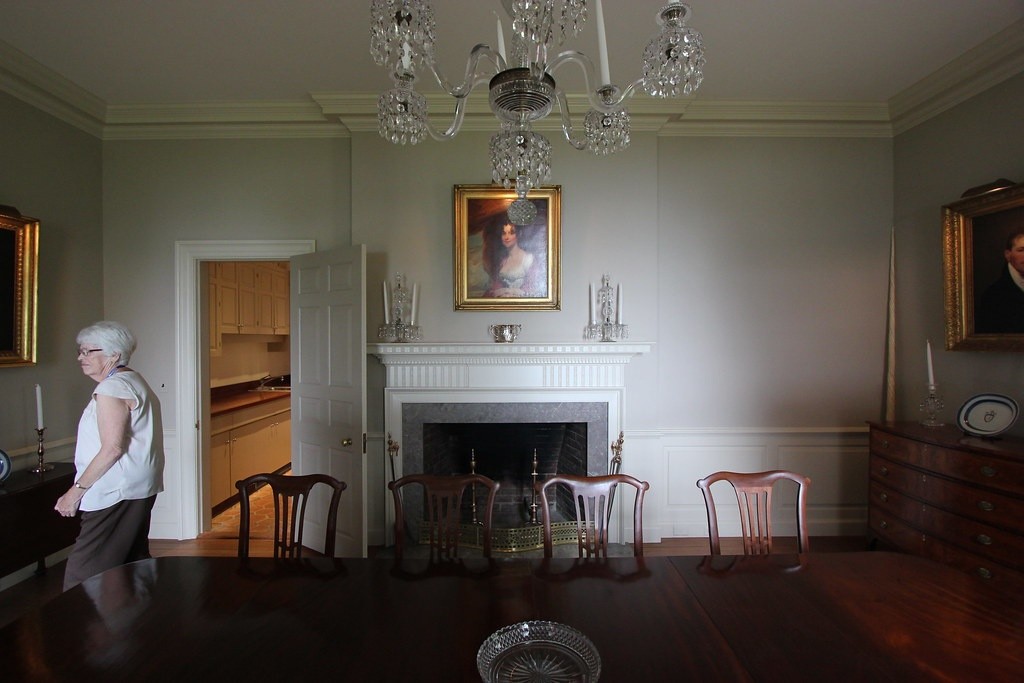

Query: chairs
[532,474,650,557]
[388,473,500,558]
[235,472,348,558]
[698,468,810,555]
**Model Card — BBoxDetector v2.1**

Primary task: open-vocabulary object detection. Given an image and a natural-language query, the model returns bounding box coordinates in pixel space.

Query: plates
[0,448,12,482]
[954,392,1020,436]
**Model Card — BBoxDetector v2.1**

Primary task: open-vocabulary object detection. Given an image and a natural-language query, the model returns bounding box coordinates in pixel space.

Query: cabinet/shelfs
[867,418,1024,572]
[208,390,291,519]
[0,461,85,593]
[208,260,290,358]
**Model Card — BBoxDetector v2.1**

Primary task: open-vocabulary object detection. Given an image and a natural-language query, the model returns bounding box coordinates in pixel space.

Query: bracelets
[54,321,165,592]
[75,482,87,491]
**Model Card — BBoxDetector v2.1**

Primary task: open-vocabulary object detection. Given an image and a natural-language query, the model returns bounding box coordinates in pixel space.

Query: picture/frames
[940,181,1024,353]
[0,210,41,368]
[452,182,563,314]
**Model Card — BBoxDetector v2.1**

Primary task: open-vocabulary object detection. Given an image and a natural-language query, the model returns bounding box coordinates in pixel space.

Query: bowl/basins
[476,620,602,683]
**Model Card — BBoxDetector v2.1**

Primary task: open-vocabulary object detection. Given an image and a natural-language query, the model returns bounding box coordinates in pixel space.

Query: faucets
[261,373,284,387]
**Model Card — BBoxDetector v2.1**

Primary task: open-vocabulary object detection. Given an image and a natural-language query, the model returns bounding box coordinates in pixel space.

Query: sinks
[248,387,291,392]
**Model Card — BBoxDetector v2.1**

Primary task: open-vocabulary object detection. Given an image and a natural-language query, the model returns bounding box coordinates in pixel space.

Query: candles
[35,384,43,432]
[591,282,596,325]
[383,281,390,325]
[616,283,623,325]
[926,339,934,385]
[411,282,417,326]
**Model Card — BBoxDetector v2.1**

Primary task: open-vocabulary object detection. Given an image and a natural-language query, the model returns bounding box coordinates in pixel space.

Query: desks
[0,554,1024,683]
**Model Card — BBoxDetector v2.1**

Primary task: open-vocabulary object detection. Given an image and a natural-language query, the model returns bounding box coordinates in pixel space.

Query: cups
[489,324,522,343]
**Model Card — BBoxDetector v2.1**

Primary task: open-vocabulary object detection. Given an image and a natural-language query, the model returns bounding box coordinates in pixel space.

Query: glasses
[77,348,103,355]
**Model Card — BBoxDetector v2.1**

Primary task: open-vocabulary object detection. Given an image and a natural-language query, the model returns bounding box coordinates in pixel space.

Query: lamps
[369,0,708,226]
